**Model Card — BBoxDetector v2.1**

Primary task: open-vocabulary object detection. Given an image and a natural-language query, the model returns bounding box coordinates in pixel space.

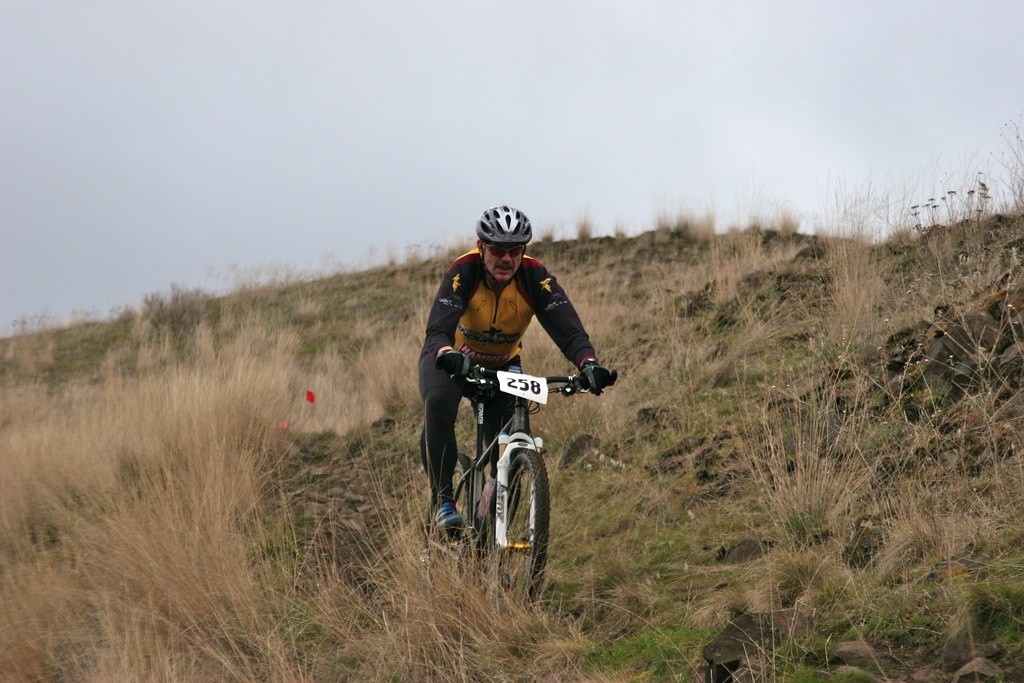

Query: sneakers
[435,501,463,528]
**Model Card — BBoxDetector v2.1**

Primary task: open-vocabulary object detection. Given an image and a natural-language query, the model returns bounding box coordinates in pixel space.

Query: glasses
[481,241,526,258]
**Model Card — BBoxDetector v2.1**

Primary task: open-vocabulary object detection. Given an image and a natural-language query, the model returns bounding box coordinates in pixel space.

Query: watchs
[581,358,599,370]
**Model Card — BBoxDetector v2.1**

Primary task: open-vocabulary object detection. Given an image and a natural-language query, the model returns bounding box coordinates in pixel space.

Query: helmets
[475,205,532,245]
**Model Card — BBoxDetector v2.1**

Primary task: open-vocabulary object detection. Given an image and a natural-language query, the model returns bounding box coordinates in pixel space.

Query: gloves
[579,356,611,396]
[434,350,477,381]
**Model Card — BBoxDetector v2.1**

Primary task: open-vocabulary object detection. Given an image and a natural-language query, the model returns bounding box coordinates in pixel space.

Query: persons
[417,204,610,539]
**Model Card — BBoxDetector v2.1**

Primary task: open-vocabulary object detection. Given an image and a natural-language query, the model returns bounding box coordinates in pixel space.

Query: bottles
[478,469,496,519]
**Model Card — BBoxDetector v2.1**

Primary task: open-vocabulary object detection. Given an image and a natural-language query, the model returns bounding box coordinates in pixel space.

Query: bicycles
[424,365,617,612]
